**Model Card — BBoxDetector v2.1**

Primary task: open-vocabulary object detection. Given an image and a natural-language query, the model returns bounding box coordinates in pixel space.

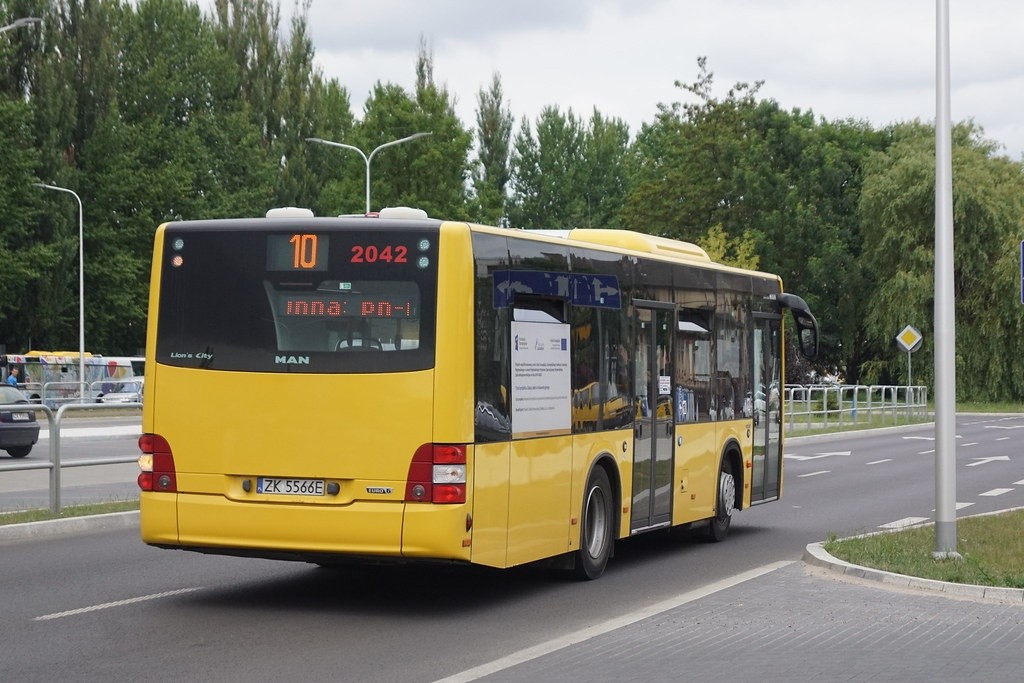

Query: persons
[6,367,19,387]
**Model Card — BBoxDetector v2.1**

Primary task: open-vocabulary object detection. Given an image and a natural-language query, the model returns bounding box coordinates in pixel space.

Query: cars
[569,380,634,430]
[0,382,41,458]
[95,378,143,403]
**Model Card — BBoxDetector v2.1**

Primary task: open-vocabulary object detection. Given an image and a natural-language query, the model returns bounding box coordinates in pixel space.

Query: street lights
[32,182,86,406]
[305,130,434,215]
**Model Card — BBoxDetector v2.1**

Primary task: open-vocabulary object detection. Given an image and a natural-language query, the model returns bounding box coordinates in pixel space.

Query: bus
[137,204,818,583]
